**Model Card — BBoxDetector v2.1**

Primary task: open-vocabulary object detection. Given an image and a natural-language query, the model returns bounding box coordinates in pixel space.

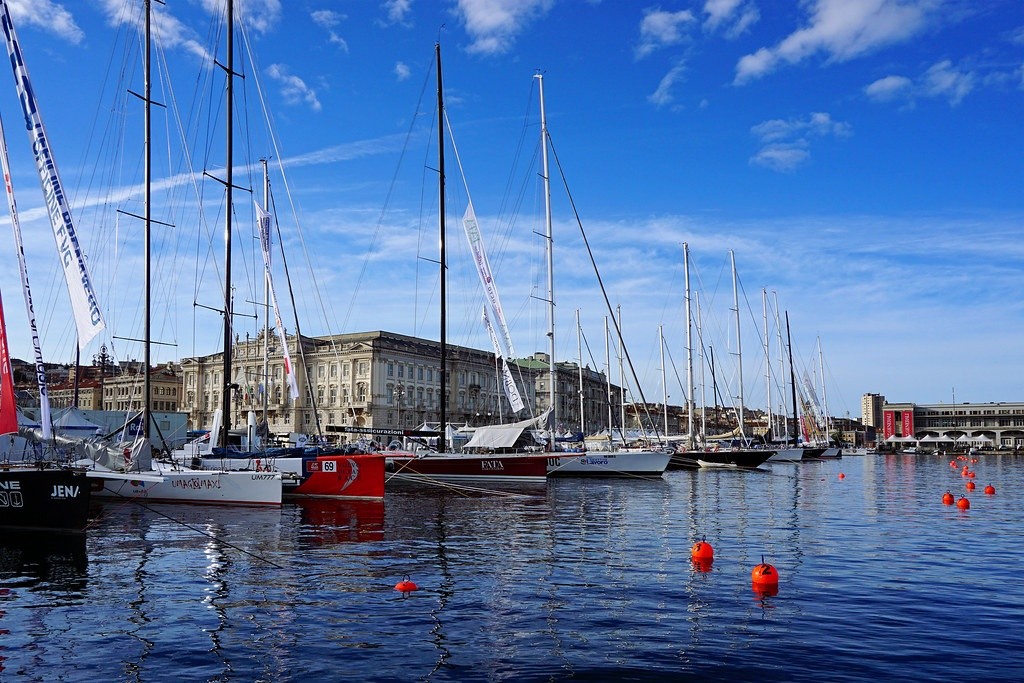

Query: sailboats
[0,1,845,542]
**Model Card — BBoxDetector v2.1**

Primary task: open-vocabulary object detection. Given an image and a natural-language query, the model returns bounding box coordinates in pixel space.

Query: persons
[633,439,644,447]
[176,445,184,450]
[161,445,169,459]
[151,444,161,459]
[94,428,104,444]
[388,445,398,454]
[351,448,361,455]
